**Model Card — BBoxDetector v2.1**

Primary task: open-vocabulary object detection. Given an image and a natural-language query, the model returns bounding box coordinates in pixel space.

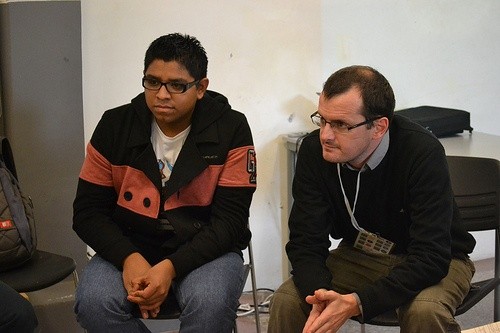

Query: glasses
[142,77,202,94]
[311,111,383,133]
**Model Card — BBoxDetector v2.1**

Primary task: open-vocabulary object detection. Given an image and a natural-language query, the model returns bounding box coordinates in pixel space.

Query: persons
[72,33,256,333]
[268,66,476,333]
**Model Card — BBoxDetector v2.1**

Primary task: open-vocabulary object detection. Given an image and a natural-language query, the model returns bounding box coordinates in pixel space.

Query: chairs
[74,232,262,333]
[349,156,500,333]
[0,137,76,293]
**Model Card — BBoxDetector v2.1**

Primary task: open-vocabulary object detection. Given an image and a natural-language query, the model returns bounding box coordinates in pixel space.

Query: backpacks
[0,160,37,270]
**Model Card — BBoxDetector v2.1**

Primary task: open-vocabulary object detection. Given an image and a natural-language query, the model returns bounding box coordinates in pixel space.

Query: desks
[279,130,500,282]
